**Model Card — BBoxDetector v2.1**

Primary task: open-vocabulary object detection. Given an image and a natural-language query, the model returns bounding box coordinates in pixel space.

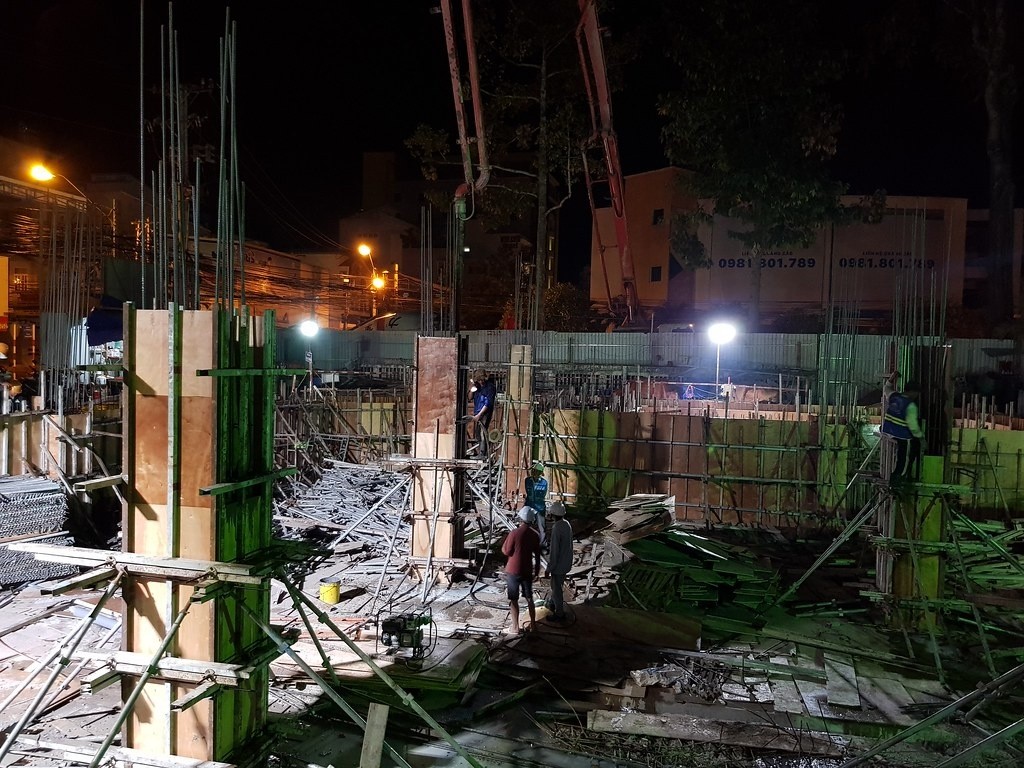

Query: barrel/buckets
[318,578,341,605]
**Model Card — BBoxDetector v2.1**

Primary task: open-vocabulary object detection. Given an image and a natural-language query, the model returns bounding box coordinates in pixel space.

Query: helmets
[519,506,534,521]
[549,502,566,516]
[532,463,544,471]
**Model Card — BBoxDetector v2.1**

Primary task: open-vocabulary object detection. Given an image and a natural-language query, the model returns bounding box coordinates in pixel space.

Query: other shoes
[470,452,485,461]
[890,474,904,485]
[546,614,565,621]
[545,603,554,611]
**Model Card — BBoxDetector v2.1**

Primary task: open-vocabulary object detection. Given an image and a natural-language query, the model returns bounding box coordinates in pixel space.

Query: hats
[475,369,487,380]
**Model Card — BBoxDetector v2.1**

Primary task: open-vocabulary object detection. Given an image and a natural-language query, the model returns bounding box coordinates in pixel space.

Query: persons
[502,507,542,634]
[0,381,26,413]
[467,369,494,460]
[524,464,547,552]
[544,501,573,627]
[883,371,925,485]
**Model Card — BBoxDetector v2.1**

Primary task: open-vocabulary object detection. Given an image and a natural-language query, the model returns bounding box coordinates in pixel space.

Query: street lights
[709,322,734,401]
[300,319,321,398]
[28,162,121,259]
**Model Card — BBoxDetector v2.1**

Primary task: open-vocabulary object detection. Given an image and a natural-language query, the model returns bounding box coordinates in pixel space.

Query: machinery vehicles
[352,309,437,333]
[439,1,702,333]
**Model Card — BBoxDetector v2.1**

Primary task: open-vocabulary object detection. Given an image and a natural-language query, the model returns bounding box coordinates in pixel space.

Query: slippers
[524,622,536,631]
[503,627,518,634]
